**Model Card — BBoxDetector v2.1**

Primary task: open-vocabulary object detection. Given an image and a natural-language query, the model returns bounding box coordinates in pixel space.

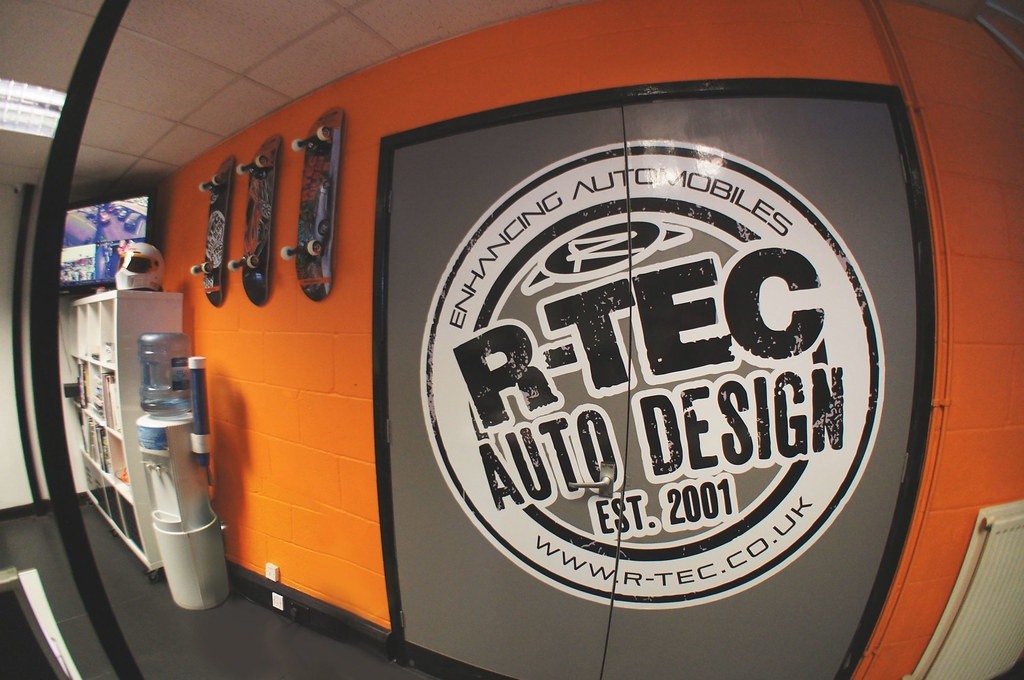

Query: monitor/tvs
[55,188,158,289]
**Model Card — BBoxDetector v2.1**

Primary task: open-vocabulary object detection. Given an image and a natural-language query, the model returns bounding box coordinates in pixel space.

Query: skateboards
[277,103,346,301]
[226,132,284,306]
[189,154,237,307]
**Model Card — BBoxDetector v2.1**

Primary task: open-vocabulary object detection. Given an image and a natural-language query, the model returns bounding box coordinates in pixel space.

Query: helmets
[115,241,164,291]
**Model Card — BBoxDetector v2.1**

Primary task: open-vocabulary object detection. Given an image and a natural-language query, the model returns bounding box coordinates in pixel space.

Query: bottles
[137,330,192,415]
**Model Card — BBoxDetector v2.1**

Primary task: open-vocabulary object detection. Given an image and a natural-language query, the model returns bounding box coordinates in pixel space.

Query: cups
[190,368,210,466]
